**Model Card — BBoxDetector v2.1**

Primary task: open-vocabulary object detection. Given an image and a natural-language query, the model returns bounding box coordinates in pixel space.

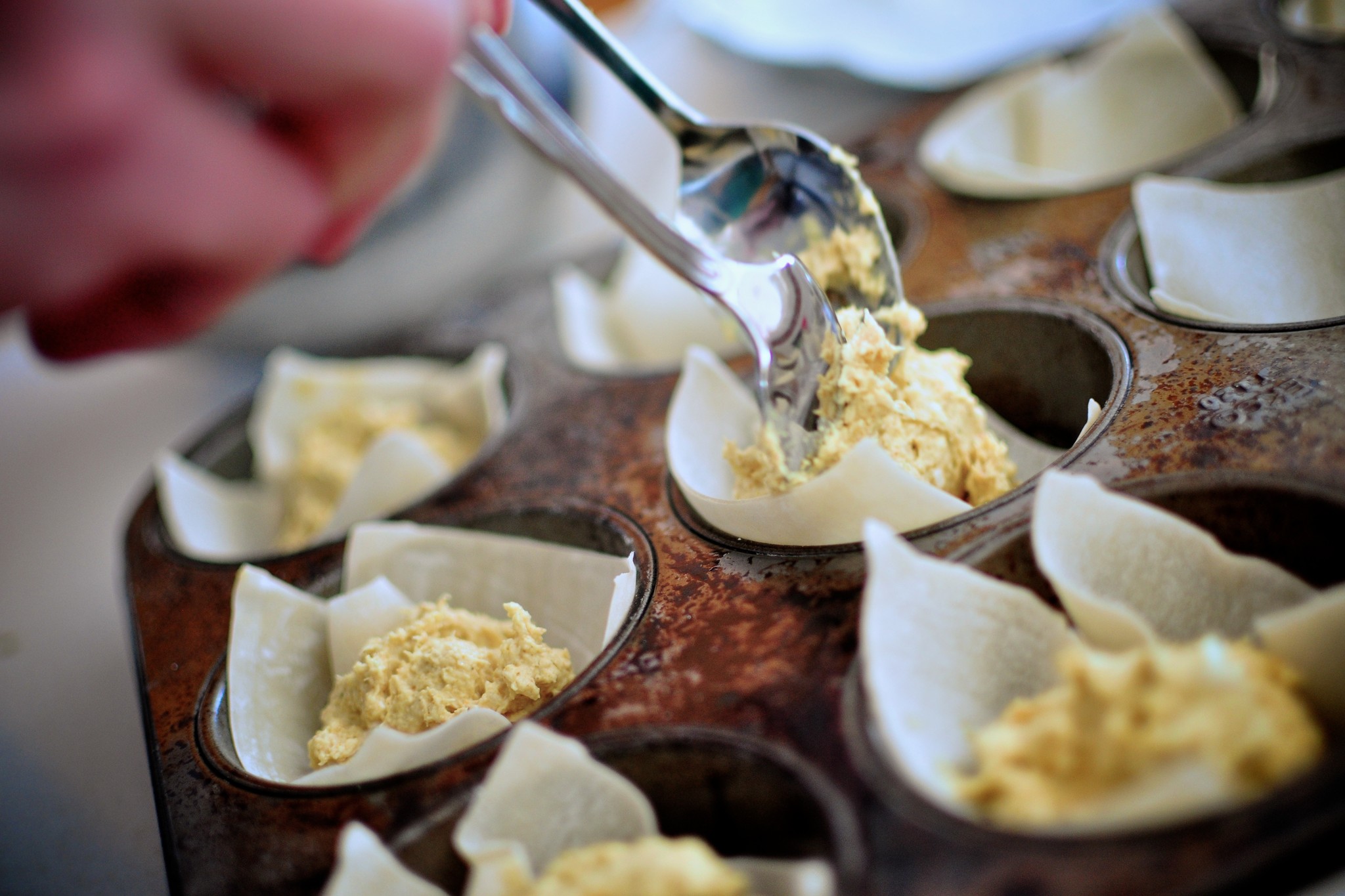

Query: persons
[0,0,683,896]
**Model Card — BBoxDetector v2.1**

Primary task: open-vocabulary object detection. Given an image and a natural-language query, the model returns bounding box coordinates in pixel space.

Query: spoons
[527,1,907,344]
[443,17,846,475]
